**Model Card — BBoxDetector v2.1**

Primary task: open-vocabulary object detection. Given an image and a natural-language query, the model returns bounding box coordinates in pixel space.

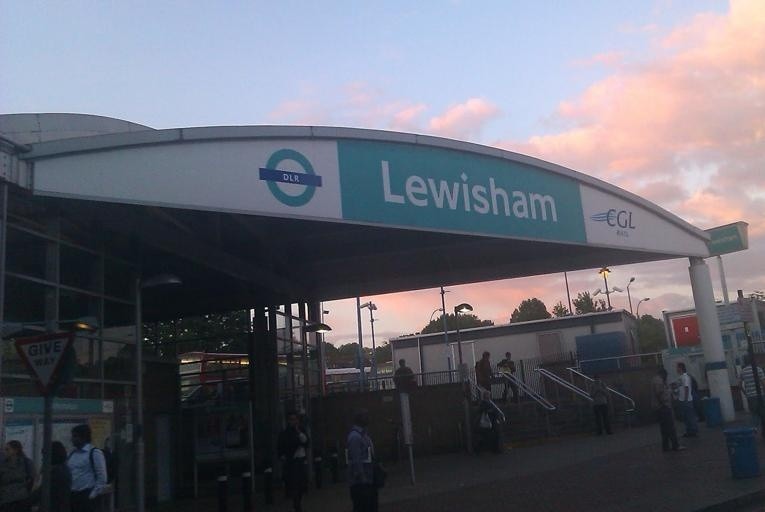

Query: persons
[473,351,493,401]
[670,360,701,438]
[650,366,687,451]
[470,390,507,455]
[0,438,37,512]
[62,423,109,512]
[735,355,765,435]
[36,439,73,511]
[278,411,311,512]
[100,435,113,483]
[393,360,418,389]
[496,351,521,403]
[341,406,391,512]
[589,371,616,434]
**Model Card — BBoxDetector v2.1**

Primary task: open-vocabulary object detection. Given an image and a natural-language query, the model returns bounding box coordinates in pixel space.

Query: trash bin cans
[701,397,723,427]
[722,427,759,478]
